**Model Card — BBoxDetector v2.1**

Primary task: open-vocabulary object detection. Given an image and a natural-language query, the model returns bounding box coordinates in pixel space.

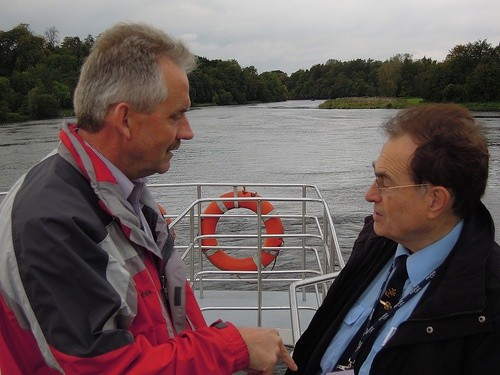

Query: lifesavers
[198,189,283,271]
[157,203,174,243]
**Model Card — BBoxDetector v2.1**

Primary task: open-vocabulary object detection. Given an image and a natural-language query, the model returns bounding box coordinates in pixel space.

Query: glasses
[376,172,432,192]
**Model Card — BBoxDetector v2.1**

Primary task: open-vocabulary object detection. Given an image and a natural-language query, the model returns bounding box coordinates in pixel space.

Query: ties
[330,254,409,375]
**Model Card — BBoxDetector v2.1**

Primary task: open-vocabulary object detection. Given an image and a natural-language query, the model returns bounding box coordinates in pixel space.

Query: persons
[285,102,500,375]
[1,23,298,375]
